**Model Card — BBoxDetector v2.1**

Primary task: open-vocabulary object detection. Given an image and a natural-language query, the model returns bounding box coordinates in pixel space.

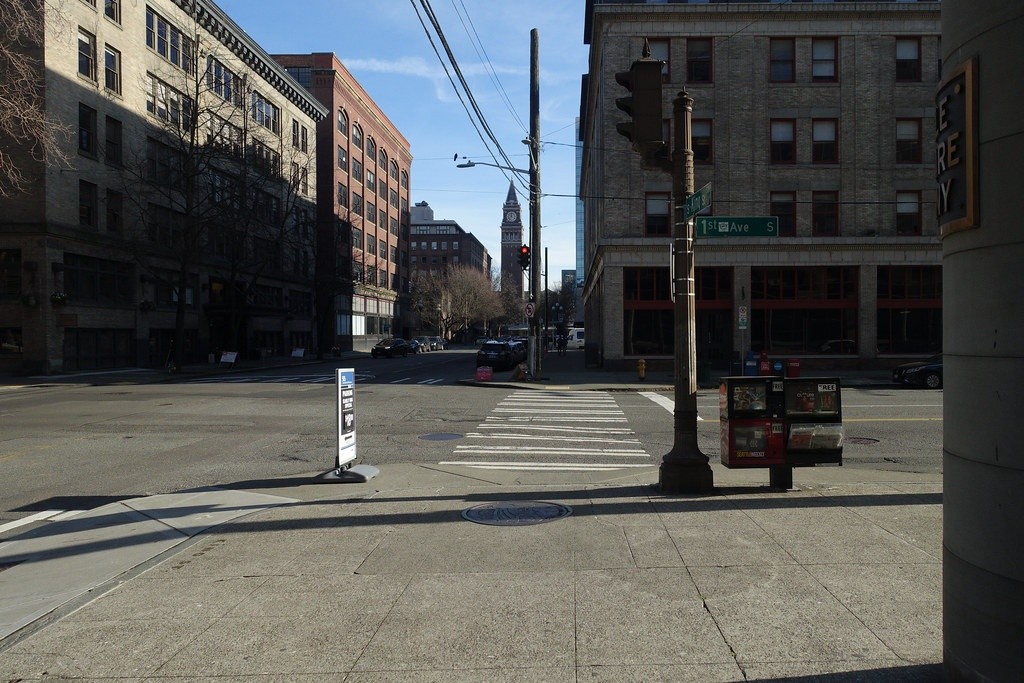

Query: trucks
[566,330,584,350]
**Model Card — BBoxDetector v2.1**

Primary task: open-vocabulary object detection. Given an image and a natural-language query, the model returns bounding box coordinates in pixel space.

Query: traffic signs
[686,182,714,221]
[695,215,779,238]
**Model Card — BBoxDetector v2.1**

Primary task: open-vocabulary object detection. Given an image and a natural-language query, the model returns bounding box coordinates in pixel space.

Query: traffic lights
[615,37,666,170]
[516,244,531,271]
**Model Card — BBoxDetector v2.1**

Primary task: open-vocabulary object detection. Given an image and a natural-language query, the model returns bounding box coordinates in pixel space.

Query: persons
[557,336,562,352]
[562,336,568,352]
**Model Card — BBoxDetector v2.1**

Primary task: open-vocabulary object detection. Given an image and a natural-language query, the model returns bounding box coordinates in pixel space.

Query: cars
[892,352,943,390]
[473,335,529,367]
[817,339,856,354]
[402,335,448,355]
[370,338,409,358]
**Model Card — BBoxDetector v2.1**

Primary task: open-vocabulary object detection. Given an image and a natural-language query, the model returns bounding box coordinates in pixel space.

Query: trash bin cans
[717,375,843,492]
[744,350,757,376]
[584,341,600,368]
[786,357,801,377]
[759,350,772,376]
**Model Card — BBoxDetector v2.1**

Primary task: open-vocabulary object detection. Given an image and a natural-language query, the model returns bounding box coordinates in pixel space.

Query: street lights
[456,27,542,383]
